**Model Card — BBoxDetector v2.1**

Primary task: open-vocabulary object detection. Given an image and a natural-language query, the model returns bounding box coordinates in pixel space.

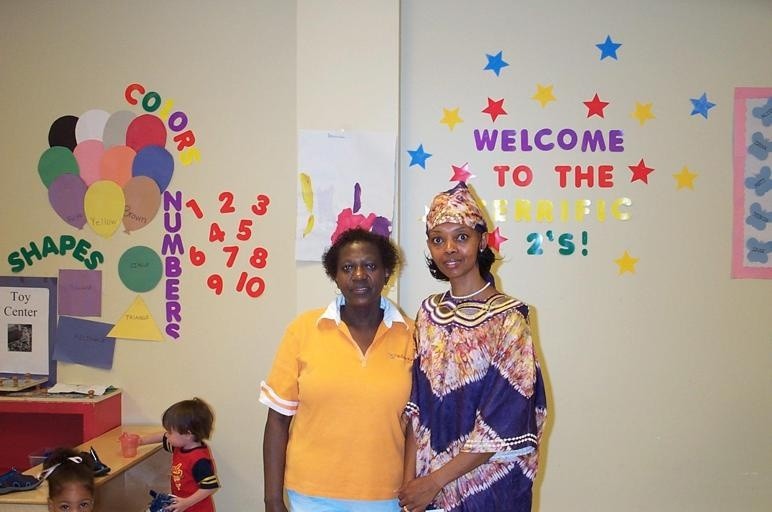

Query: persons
[257,226,415,512]
[138,396,220,512]
[398,179,548,512]
[42,446,96,512]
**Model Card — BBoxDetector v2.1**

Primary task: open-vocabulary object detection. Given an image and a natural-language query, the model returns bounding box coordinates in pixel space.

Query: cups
[118,434,141,458]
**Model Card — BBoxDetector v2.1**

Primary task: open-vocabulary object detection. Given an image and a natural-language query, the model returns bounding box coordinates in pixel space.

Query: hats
[425,180,488,234]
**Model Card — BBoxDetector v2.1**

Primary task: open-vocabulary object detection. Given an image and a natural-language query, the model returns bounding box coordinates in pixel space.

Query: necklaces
[450,283,491,299]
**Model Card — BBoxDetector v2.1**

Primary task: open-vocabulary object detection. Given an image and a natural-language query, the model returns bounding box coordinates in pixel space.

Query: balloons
[117,246,164,294]
[36,109,175,242]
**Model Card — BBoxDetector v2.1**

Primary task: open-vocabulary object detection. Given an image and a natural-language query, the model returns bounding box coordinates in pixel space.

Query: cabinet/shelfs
[0,388,173,511]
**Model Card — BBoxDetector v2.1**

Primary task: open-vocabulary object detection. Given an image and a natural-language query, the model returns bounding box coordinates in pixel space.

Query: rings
[403,506,409,512]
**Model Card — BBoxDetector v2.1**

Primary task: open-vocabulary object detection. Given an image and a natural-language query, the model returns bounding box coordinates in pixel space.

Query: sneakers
[0,470,43,495]
[79,446,111,478]
